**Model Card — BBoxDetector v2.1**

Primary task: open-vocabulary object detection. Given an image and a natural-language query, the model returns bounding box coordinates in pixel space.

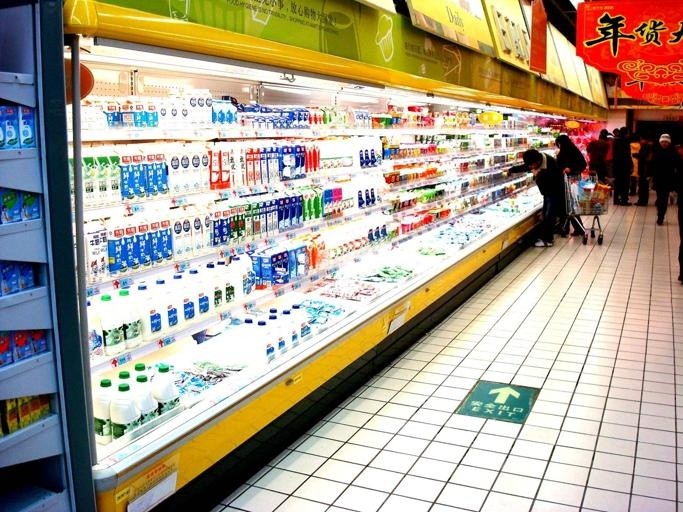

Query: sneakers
[535,238,553,247]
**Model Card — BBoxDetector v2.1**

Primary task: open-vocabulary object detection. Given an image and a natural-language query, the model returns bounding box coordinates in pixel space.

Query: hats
[659,133,672,144]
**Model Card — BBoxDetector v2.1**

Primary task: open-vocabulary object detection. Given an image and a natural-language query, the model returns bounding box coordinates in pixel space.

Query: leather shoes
[613,200,649,206]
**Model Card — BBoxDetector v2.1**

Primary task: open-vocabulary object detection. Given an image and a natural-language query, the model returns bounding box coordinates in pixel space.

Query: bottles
[67,253,315,455]
[156,84,238,132]
[305,91,439,272]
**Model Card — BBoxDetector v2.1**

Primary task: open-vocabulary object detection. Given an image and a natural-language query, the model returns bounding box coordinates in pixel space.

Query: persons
[505,125,683,283]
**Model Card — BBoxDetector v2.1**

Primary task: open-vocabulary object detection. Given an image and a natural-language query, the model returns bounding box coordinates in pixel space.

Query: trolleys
[561,167,612,245]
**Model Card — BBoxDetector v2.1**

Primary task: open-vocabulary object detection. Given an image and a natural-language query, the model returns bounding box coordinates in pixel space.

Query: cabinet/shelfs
[62,92,571,477]
[0,65,73,511]
